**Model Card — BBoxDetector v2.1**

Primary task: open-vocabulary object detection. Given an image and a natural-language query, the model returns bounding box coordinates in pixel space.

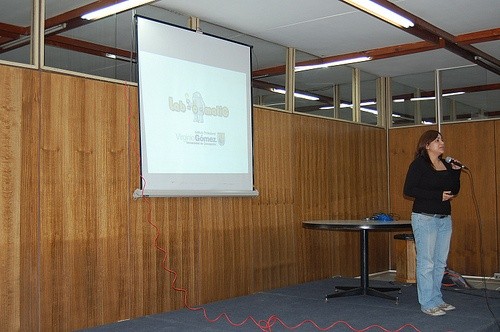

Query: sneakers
[421,307,446,316]
[438,303,455,310]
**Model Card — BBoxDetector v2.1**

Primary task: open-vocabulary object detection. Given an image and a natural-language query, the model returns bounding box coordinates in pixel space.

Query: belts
[412,210,448,219]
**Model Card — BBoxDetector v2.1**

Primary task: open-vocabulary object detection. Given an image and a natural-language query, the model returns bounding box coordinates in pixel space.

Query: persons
[403,129,463,316]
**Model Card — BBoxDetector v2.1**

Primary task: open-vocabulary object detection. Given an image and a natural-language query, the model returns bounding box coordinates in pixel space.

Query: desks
[302,219,413,302]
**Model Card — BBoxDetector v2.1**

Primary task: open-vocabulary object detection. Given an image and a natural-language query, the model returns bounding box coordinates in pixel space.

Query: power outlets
[494,273,500,281]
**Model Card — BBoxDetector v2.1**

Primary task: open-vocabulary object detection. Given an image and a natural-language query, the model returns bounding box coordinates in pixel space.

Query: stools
[393,234,418,254]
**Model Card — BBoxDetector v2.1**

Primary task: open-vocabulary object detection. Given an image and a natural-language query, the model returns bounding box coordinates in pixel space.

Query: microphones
[445,157,468,170]
